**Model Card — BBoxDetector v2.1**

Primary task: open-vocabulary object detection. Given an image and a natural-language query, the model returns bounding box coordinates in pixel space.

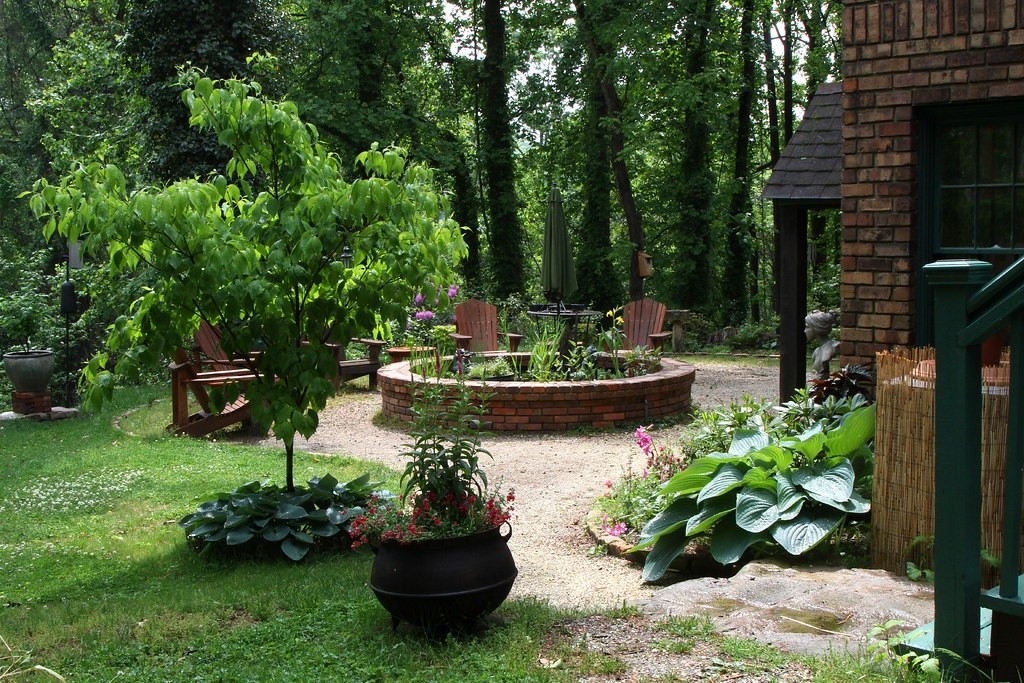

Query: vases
[366,520,519,633]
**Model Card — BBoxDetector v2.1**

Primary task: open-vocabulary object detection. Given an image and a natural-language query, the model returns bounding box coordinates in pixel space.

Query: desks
[527,309,602,351]
[386,346,437,363]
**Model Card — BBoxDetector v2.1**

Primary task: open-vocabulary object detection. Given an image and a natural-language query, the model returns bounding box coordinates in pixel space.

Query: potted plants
[0,296,55,392]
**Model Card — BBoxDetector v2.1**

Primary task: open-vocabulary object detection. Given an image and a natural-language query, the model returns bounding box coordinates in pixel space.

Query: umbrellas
[540,184,576,315]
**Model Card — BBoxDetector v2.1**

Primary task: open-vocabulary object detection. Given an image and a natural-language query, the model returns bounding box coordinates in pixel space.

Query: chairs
[168,347,281,439]
[447,297,525,354]
[196,321,266,377]
[324,328,387,392]
[597,297,673,356]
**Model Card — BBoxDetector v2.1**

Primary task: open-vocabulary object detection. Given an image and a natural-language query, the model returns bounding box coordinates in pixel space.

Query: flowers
[340,348,518,550]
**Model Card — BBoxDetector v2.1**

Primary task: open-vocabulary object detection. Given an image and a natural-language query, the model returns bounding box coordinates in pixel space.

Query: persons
[804,310,840,374]
